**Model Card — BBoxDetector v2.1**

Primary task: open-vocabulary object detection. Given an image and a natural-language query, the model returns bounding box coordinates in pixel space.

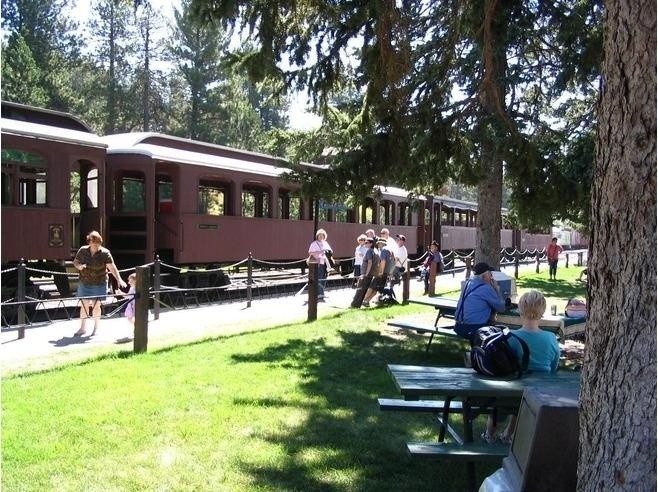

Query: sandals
[481,431,513,445]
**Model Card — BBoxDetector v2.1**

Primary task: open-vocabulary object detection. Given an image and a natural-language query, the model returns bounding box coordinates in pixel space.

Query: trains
[0,98,554,327]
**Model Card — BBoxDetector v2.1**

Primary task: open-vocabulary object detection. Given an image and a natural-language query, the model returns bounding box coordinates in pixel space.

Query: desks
[388,368,588,492]
[404,294,587,353]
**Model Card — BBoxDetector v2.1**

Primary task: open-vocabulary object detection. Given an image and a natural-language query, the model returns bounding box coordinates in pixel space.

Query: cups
[551,304,557,316]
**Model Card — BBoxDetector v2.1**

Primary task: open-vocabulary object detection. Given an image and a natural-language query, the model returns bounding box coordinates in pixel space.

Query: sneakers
[74,329,94,339]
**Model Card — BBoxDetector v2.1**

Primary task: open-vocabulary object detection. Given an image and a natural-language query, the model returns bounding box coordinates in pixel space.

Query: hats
[474,262,495,274]
[375,238,387,245]
[397,234,405,241]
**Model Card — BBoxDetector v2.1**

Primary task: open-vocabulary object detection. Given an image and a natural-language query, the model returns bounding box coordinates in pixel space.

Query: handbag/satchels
[500,298,520,315]
[323,250,337,272]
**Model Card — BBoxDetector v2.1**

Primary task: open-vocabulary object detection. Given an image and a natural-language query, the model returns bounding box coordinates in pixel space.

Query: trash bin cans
[478,387,580,492]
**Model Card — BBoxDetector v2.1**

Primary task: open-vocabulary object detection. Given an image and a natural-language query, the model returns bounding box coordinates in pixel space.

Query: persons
[454,262,506,347]
[576,263,588,280]
[73,231,127,339]
[116,273,136,339]
[481,290,561,444]
[306,227,446,309]
[547,237,563,281]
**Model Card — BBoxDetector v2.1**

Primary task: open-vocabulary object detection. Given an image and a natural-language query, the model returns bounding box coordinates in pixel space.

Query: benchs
[370,398,517,463]
[385,317,473,343]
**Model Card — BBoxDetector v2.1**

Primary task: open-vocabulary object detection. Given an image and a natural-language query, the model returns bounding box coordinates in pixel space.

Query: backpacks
[470,324,529,381]
[371,254,381,276]
